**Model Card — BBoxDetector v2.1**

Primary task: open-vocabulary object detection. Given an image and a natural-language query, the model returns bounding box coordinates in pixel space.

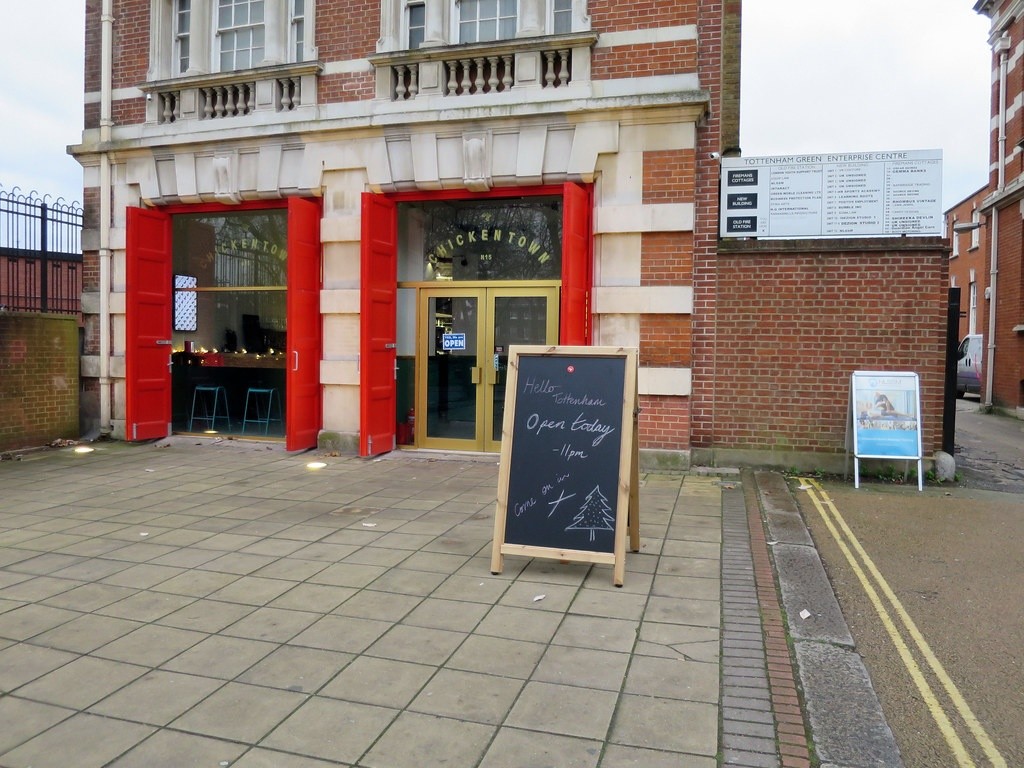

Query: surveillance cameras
[709,152,720,160]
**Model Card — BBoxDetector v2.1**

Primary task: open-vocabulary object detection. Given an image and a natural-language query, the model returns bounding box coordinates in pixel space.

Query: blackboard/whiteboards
[492,344,640,565]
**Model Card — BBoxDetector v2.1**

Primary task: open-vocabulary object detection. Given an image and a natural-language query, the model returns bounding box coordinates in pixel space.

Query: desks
[172,352,286,436]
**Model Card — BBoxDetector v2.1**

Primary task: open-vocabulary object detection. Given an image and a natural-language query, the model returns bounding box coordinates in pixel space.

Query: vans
[956,334,983,398]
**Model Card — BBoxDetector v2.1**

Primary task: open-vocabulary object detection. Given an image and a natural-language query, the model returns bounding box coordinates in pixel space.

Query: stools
[188,385,231,432]
[241,387,283,436]
